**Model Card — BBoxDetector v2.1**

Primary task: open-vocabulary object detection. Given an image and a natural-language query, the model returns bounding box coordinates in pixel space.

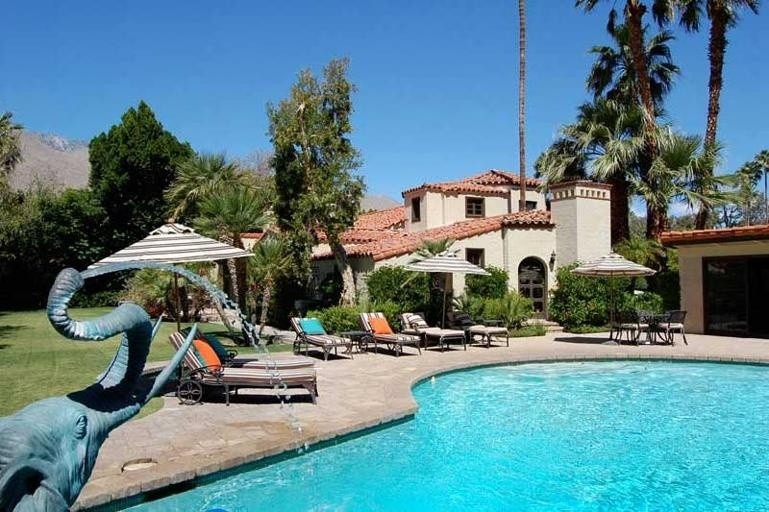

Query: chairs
[447,312,509,349]
[398,312,466,353]
[360,312,422,357]
[613,310,688,347]
[168,327,319,406]
[291,318,354,361]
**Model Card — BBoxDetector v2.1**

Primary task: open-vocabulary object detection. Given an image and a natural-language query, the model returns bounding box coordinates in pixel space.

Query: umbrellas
[402,248,495,329]
[84,214,257,398]
[570,251,659,339]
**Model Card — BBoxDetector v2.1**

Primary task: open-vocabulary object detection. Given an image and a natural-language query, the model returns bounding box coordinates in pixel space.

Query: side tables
[340,331,371,355]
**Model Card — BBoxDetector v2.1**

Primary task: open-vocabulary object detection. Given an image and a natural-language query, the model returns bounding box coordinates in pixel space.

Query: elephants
[0,267,199,512]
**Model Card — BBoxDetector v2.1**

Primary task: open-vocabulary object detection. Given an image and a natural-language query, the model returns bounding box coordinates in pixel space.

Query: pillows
[454,314,473,325]
[298,320,325,335]
[368,318,391,334]
[406,315,429,328]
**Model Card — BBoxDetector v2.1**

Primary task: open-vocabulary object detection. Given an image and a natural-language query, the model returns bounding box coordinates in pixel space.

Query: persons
[319,271,335,300]
